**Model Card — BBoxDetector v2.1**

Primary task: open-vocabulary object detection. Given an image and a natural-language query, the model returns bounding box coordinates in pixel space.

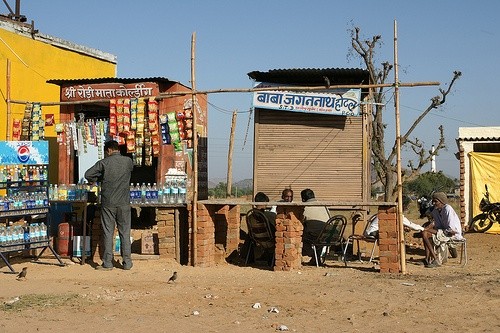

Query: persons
[252,189,332,265]
[85,139,133,270]
[365,192,462,268]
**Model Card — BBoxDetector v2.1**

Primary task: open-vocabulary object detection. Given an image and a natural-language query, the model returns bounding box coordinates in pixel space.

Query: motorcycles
[469,184,500,233]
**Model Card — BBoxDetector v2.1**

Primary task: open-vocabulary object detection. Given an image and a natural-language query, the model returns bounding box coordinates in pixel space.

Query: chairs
[244,209,275,269]
[434,241,468,267]
[302,213,378,269]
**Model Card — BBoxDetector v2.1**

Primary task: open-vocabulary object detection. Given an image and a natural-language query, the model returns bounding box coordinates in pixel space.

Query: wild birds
[168,271,179,284]
[16,267,28,281]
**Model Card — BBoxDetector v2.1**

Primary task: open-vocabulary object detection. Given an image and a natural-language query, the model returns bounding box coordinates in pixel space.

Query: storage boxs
[114,237,133,256]
[73,235,91,257]
[141,233,159,255]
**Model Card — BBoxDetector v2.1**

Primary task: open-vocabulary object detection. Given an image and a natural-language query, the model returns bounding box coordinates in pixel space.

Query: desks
[193,200,401,274]
[48,199,187,265]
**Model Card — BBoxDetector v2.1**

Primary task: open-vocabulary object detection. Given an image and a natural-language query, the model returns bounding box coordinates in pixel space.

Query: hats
[433,192,448,204]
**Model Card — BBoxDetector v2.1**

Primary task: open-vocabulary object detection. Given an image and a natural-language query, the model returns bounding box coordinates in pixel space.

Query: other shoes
[414,258,429,265]
[425,257,443,268]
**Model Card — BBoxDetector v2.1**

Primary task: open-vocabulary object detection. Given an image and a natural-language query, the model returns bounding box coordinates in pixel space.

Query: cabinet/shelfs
[0,180,66,273]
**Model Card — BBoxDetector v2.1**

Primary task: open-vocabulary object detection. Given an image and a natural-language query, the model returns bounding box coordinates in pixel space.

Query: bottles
[141,183,147,205]
[49,182,102,204]
[170,180,179,204]
[146,183,152,204]
[0,187,48,217]
[30,222,48,248]
[178,180,187,204]
[158,182,163,204]
[163,180,170,205]
[129,182,135,204]
[0,164,48,187]
[135,183,142,204]
[152,183,158,204]
[0,220,29,252]
